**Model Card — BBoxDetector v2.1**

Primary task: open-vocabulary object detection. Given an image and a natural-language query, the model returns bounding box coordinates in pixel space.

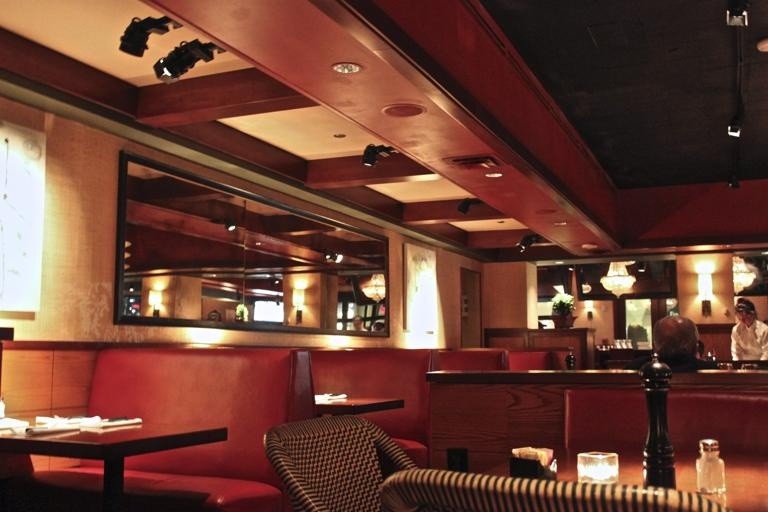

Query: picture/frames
[403,241,438,335]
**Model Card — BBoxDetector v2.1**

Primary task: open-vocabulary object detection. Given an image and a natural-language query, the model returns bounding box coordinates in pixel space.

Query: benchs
[0,326,768,512]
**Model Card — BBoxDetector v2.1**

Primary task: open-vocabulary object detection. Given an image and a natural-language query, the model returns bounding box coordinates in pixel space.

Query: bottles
[696,439,726,495]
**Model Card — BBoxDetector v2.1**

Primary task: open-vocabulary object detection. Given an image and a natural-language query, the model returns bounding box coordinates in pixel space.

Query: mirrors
[113,148,392,342]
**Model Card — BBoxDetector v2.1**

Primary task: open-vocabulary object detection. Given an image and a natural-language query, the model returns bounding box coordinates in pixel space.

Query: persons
[727,296,767,369]
[622,317,717,373]
[354,315,367,331]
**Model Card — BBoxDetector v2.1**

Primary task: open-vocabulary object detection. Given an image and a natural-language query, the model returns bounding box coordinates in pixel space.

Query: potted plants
[550,296,578,329]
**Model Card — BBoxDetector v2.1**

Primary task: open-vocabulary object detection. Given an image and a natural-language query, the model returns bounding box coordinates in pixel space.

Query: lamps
[323,245,345,263]
[599,261,637,300]
[516,233,543,254]
[221,215,237,233]
[118,15,222,85]
[458,196,482,214]
[360,141,399,166]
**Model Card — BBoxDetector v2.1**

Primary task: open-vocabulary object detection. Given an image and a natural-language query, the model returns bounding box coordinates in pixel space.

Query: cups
[576,450,620,485]
